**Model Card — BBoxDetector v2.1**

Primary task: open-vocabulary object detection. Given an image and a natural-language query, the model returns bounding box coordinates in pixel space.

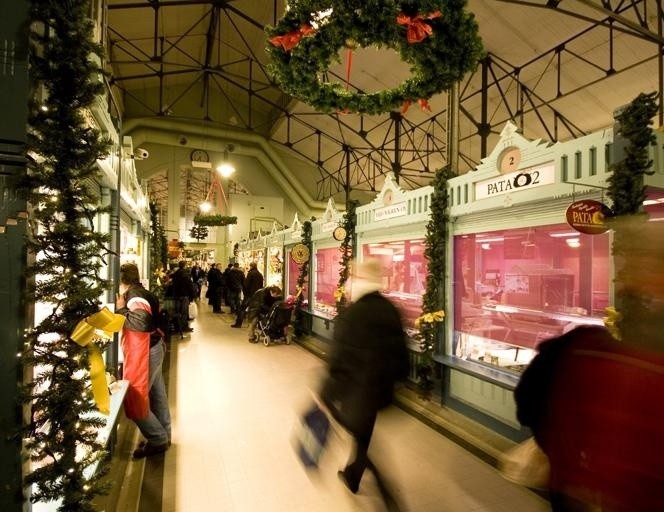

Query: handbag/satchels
[305,410,330,445]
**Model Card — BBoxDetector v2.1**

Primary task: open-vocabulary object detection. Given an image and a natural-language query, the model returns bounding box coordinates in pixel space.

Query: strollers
[253,301,294,345]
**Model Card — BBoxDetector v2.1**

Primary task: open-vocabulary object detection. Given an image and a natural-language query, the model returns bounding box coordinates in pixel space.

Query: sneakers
[299,445,316,467]
[134,440,172,456]
[184,327,193,332]
[231,324,242,328]
[338,470,358,494]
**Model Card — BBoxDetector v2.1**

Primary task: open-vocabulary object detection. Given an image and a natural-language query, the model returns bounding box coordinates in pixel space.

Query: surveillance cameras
[125,148,149,161]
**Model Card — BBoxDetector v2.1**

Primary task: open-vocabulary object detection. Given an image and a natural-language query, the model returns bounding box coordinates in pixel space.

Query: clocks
[190,149,209,162]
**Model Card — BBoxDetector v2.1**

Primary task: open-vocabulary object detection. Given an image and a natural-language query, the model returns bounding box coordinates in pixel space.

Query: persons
[320,259,412,512]
[502,270,664,512]
[170,258,282,344]
[115,263,177,459]
